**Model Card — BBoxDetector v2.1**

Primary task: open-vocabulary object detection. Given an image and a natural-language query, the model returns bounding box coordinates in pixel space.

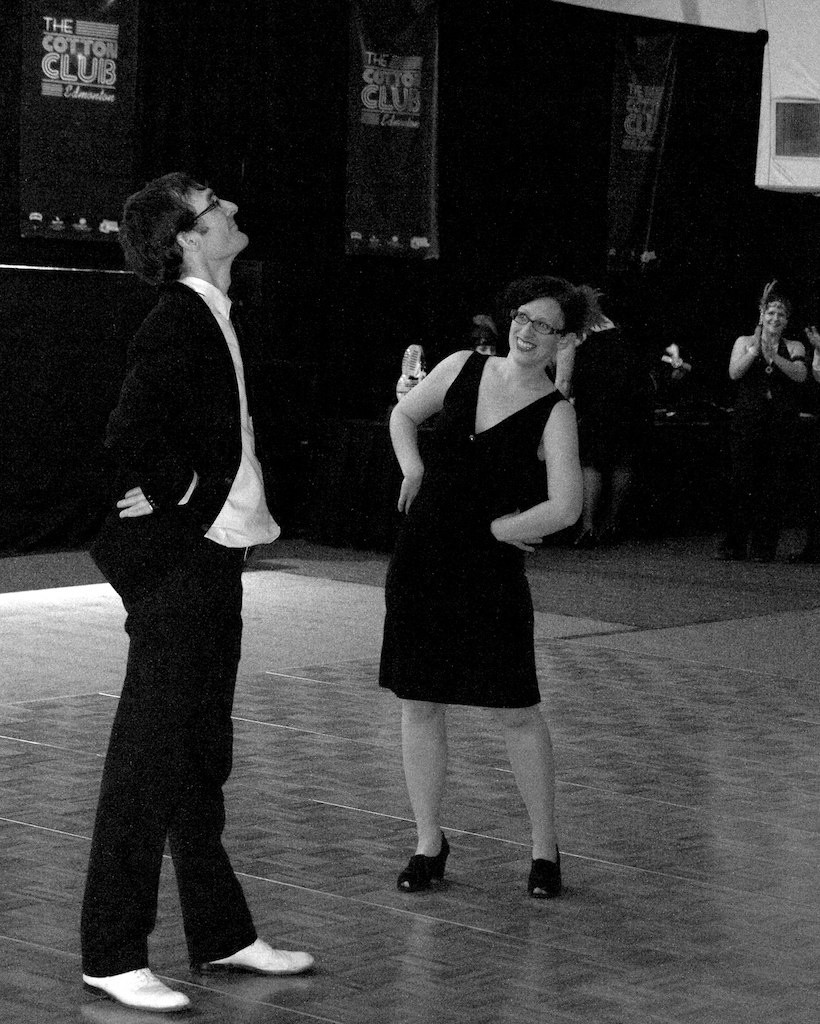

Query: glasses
[509,309,563,335]
[190,197,224,223]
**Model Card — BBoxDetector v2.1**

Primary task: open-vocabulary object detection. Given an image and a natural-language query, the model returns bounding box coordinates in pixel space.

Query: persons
[378,277,605,900]
[545,341,631,547]
[712,294,820,561]
[78,172,316,1011]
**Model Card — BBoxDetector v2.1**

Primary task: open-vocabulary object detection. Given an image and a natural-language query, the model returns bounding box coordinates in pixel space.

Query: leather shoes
[200,937,316,976]
[82,968,194,1012]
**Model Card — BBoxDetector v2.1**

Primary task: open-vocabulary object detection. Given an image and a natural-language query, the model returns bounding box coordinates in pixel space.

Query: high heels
[397,830,450,891]
[528,843,562,898]
